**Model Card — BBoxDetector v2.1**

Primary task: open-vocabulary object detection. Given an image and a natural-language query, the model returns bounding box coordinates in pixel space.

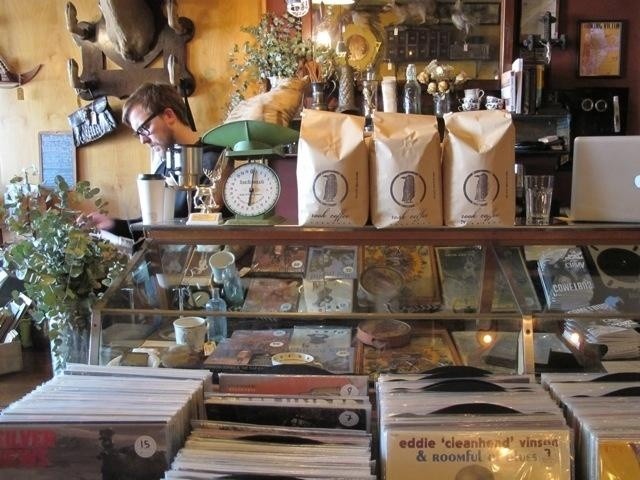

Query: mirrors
[300,1,515,94]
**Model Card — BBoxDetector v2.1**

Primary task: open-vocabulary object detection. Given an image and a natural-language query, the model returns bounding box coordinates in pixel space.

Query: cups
[208,251,239,284]
[521,171,557,226]
[269,349,324,371]
[135,172,177,227]
[456,87,505,112]
[172,315,212,355]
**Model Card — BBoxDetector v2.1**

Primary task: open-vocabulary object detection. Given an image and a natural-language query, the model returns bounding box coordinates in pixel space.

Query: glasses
[134,113,158,140]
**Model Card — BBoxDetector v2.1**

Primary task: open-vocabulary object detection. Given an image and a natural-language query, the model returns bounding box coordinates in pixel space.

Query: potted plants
[1,164,131,381]
[227,12,327,108]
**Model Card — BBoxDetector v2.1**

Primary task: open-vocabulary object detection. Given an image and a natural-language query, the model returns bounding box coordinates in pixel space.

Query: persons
[76,81,221,253]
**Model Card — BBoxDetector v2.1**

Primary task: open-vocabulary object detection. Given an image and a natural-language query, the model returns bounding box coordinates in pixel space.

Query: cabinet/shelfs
[85,212,639,379]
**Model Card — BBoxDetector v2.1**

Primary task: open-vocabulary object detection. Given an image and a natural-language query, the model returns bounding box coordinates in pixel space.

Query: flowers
[418,59,472,97]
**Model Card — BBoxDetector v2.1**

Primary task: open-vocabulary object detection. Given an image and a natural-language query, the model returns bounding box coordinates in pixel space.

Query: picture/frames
[36,130,79,192]
[576,18,627,80]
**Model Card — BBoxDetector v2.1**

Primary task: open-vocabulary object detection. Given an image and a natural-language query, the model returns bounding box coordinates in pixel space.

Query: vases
[432,94,453,116]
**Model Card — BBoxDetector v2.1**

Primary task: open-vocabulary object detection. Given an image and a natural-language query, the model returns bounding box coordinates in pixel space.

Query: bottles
[402,62,423,115]
[204,287,230,344]
[361,62,380,118]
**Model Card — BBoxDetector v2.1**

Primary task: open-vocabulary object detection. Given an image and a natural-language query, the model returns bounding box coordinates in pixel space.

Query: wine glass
[164,143,205,225]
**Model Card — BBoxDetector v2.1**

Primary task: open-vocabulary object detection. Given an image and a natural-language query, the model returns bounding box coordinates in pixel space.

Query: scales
[201,120,299,226]
